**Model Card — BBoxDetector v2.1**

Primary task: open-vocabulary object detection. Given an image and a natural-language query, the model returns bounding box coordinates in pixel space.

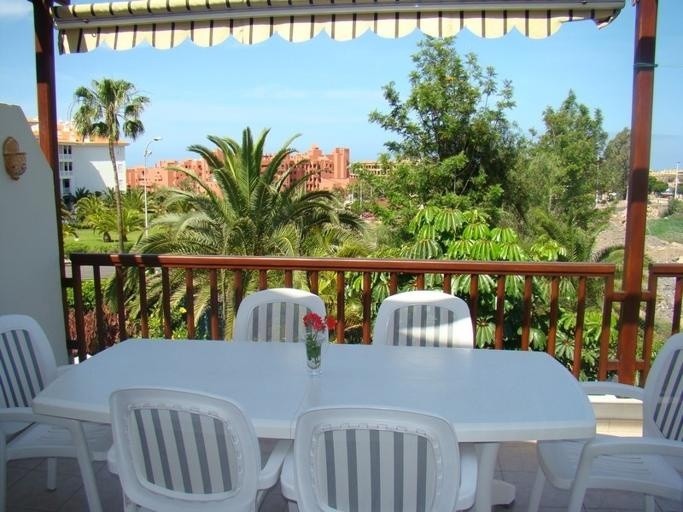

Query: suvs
[609,192,616,201]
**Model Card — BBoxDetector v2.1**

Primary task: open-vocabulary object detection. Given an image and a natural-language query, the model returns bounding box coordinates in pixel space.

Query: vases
[304,333,325,375]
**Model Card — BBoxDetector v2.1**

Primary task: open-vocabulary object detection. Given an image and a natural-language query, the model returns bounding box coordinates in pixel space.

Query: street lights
[144,137,163,236]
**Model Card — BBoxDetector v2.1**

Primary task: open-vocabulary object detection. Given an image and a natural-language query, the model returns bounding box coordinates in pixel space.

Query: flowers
[300,311,336,332]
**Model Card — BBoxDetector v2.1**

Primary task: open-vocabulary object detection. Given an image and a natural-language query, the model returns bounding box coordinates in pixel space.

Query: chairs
[524,330,683,511]
[0,312,117,512]
[287,405,478,510]
[105,385,288,512]
[234,286,331,346]
[372,289,477,350]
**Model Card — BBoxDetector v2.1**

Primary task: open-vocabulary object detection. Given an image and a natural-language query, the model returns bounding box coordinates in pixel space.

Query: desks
[29,336,600,510]
